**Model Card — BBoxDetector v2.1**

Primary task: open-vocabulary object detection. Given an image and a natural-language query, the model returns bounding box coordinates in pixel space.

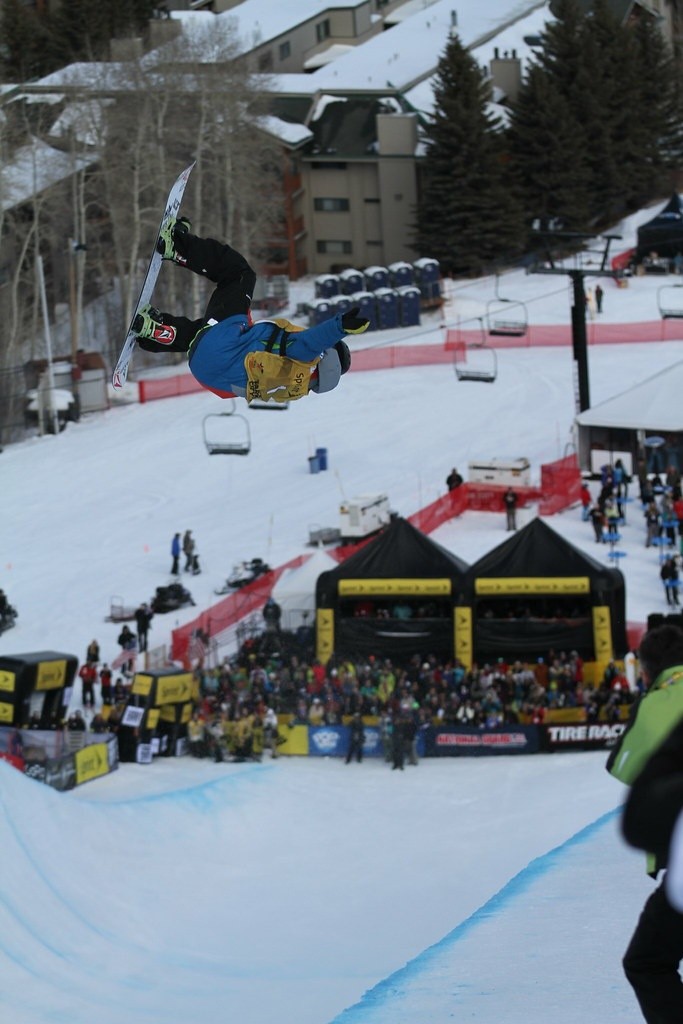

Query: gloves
[343,307,370,334]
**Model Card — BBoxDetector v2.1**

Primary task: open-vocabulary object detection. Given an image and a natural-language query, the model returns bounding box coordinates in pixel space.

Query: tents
[575,361,683,471]
[638,192,683,259]
[313,517,626,667]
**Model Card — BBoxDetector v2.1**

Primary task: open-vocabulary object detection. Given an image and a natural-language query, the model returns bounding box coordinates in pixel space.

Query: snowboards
[113,159,196,389]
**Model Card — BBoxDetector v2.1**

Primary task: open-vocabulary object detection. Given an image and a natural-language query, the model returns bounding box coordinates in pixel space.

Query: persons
[666,467,681,502]
[603,625,683,1024]
[183,530,194,571]
[447,468,462,492]
[643,483,683,547]
[587,285,603,320]
[660,558,679,604]
[171,534,180,575]
[119,626,137,673]
[135,604,153,653]
[9,640,130,755]
[192,650,644,770]
[581,459,625,543]
[263,597,281,631]
[636,458,647,497]
[503,487,517,530]
[132,219,371,405]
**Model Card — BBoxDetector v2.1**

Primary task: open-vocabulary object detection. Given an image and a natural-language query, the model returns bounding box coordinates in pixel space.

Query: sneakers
[132,305,161,338]
[156,216,191,253]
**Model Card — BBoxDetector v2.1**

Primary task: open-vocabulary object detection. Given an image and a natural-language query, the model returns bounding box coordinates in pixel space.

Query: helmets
[335,338,351,376]
[638,622,683,687]
